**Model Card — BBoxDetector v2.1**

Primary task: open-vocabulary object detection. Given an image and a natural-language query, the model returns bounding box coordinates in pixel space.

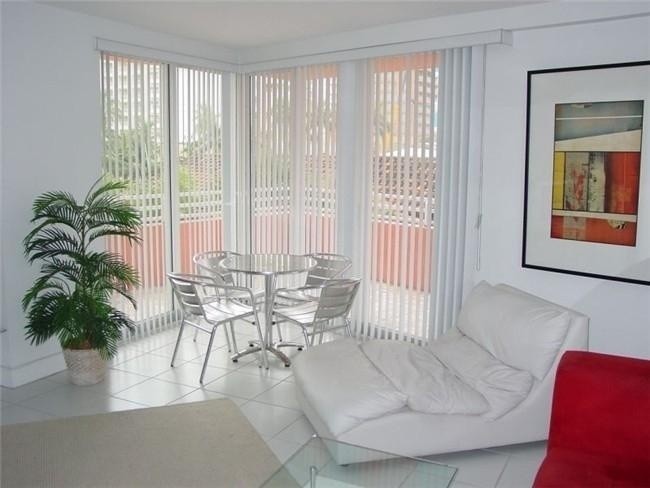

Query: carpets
[0,396,302,488]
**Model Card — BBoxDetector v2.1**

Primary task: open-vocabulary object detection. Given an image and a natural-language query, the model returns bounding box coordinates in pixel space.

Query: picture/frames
[521,60,650,286]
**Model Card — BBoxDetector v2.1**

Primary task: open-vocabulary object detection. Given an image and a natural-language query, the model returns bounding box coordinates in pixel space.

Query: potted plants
[22,171,145,388]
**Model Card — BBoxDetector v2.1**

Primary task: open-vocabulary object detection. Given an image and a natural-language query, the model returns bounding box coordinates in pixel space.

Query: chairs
[289,279,590,467]
[164,250,361,382]
[533,351,650,488]
